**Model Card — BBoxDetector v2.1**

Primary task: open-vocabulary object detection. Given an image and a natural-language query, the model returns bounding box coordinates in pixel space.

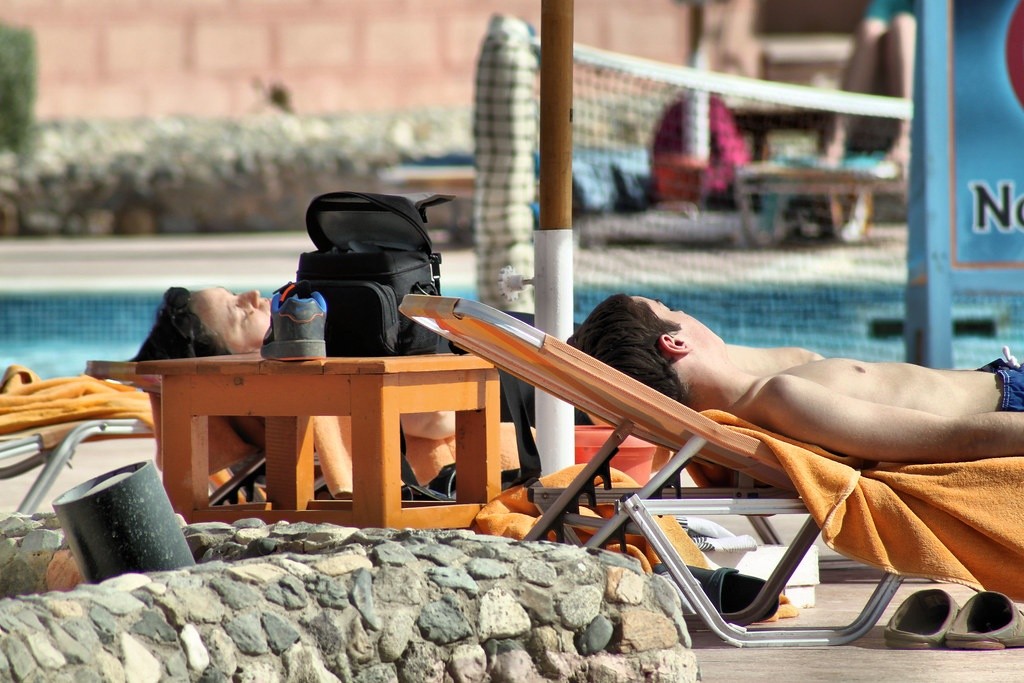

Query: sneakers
[261,280,327,360]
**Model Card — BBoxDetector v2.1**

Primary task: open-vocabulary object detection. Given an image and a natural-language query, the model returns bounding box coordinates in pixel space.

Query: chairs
[84,360,948,585]
[397,294,1024,649]
[0,364,266,515]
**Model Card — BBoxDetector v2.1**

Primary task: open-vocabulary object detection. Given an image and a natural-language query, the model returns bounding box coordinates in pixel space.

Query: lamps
[51,459,196,584]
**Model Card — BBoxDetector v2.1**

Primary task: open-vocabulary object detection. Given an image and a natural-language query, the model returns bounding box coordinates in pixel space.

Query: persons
[566,294,1023,463]
[134,286,457,440]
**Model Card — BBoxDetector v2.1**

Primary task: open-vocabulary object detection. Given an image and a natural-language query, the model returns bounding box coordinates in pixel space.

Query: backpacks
[297,190,457,354]
[652,97,748,208]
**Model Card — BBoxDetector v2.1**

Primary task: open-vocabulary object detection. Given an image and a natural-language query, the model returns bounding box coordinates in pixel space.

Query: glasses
[164,287,203,357]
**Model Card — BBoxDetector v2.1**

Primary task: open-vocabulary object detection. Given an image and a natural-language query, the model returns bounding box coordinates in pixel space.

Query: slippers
[653,565,779,627]
[884,589,1024,650]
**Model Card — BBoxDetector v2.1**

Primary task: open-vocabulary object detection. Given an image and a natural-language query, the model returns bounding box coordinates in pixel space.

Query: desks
[734,158,904,250]
[135,353,501,529]
[381,163,540,249]
